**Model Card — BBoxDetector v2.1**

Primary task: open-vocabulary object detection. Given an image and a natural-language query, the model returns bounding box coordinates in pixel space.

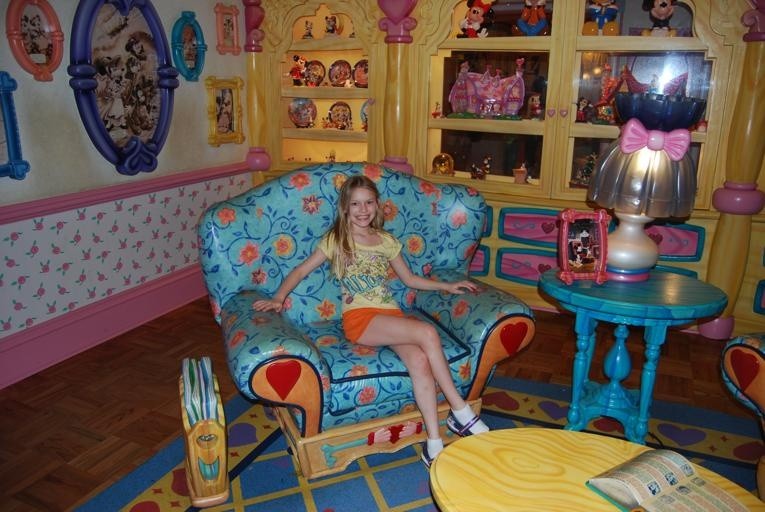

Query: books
[582,447,749,512]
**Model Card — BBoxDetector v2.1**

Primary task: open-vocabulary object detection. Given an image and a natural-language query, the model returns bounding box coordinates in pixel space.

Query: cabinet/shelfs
[412,0,753,221]
[273,34,385,170]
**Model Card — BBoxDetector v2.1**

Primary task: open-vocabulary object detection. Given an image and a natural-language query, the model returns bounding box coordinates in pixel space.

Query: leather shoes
[446,409,491,437]
[420,441,433,469]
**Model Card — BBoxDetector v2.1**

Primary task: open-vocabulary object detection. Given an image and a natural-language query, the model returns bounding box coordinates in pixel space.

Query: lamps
[587,116,699,289]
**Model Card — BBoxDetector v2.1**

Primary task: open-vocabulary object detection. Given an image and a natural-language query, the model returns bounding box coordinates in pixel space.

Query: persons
[251,175,495,469]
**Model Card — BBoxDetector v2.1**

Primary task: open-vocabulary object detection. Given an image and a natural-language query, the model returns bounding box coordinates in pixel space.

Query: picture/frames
[558,206,610,284]
[4,0,65,81]
[66,0,182,177]
[213,3,244,55]
[1,70,31,181]
[169,9,206,84]
[205,76,245,148]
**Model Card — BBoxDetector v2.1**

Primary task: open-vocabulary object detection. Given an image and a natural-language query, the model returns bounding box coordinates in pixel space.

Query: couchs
[198,161,541,481]
[718,326,765,433]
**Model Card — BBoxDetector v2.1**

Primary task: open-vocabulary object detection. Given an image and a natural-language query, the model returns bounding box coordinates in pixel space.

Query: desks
[536,268,728,448]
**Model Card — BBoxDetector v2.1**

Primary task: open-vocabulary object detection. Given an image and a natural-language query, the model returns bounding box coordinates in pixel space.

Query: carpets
[72,374,765,512]
